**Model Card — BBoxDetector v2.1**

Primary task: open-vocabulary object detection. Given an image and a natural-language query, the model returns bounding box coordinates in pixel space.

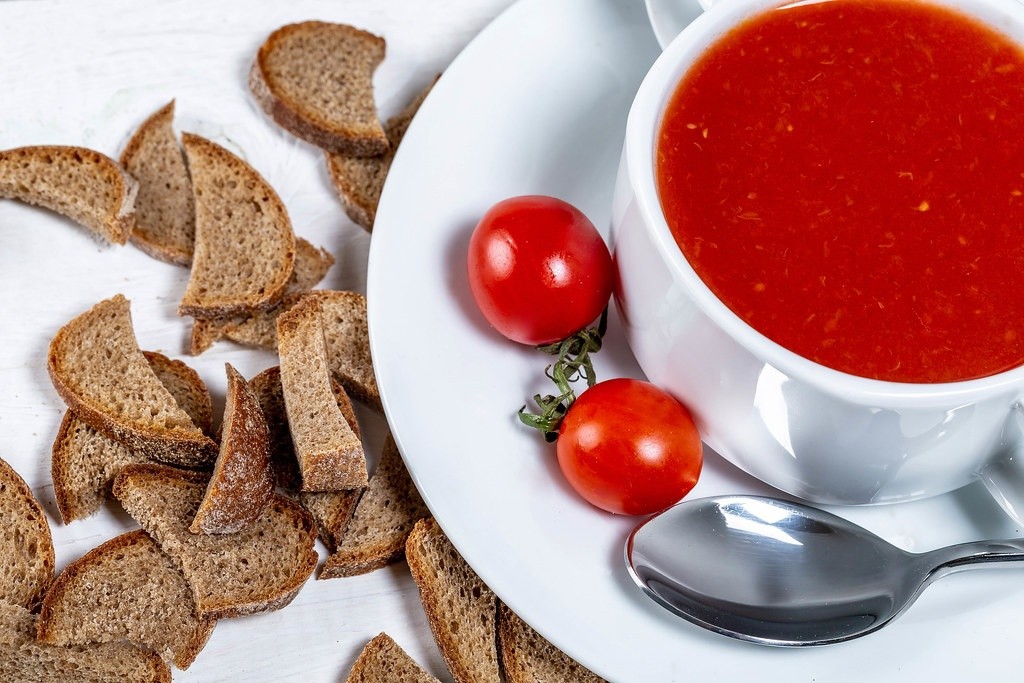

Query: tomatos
[556,377,702,518]
[467,195,615,347]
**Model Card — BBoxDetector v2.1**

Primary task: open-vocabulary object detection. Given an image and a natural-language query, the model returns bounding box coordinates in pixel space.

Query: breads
[0,16,620,683]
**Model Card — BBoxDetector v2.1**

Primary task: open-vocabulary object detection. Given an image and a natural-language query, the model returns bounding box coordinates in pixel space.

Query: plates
[365,0,1024,683]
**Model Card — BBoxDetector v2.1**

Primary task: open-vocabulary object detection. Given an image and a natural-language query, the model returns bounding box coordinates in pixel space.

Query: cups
[604,0,1024,505]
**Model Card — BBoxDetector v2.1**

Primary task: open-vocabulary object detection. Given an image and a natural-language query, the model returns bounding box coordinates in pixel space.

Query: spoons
[624,495,1024,649]
